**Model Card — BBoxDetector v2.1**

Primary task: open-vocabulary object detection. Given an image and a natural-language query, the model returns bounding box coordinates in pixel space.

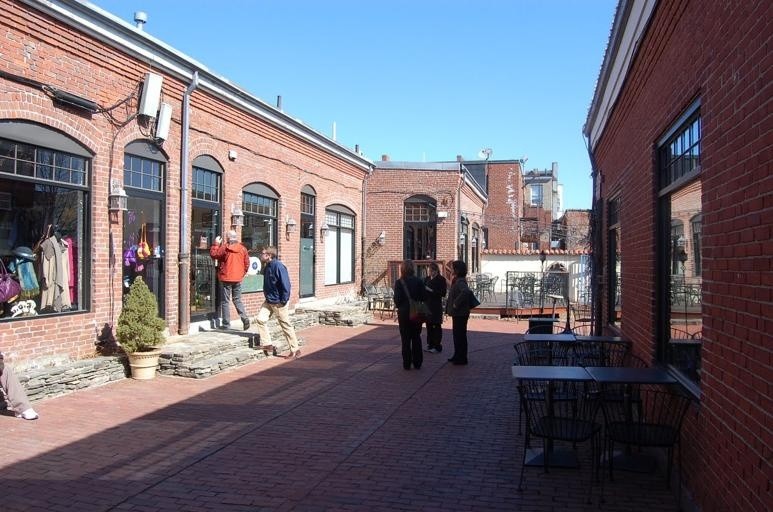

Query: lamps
[319,221,331,237]
[284,214,297,239]
[376,231,386,245]
[229,206,246,227]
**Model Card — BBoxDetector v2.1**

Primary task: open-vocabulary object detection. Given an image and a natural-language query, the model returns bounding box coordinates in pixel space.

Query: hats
[13,246,37,262]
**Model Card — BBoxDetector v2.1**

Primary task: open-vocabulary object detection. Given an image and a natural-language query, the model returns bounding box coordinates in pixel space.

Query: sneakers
[423,344,438,353]
[217,326,229,330]
[244,319,250,330]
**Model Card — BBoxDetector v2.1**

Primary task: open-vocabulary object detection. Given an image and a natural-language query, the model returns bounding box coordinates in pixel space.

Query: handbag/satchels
[0,273,21,302]
[409,299,432,322]
[459,290,480,312]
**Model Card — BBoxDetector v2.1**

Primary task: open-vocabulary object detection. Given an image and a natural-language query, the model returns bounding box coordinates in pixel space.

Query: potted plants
[115,272,167,381]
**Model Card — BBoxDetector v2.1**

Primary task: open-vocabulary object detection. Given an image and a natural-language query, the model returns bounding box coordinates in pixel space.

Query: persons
[393,259,425,369]
[210,230,250,331]
[0,351,38,420]
[445,260,470,366]
[40,231,72,312]
[423,263,447,353]
[253,247,301,359]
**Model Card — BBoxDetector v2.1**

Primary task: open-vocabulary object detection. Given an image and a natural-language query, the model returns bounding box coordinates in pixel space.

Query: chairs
[511,302,693,512]
[361,269,571,325]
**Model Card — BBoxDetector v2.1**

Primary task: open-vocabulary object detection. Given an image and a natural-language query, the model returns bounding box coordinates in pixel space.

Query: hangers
[50,228,64,245]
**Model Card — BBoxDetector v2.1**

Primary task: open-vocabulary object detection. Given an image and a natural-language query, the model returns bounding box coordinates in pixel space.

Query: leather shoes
[254,345,273,352]
[284,350,301,359]
